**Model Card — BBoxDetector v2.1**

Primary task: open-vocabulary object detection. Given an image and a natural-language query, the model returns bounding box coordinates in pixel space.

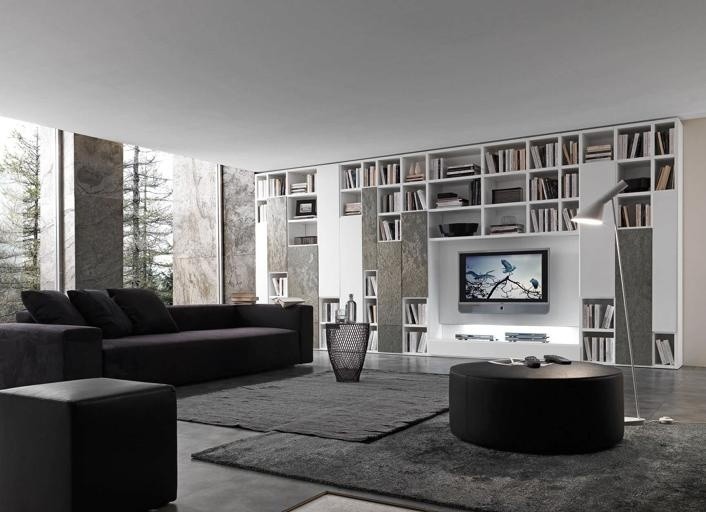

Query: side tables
[325,323,370,383]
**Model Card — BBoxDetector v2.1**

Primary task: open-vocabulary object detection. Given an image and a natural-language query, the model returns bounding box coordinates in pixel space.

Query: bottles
[344,294,357,324]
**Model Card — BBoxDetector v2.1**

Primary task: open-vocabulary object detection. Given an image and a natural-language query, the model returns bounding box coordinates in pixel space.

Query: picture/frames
[295,199,316,216]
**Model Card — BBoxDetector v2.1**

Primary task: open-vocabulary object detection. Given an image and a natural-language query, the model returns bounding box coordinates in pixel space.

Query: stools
[0,378,178,512]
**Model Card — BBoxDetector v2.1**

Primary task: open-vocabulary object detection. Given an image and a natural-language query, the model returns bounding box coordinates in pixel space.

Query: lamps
[571,180,647,426]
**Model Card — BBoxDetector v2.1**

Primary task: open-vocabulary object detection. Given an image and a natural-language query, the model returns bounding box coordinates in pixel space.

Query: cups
[334,309,347,324]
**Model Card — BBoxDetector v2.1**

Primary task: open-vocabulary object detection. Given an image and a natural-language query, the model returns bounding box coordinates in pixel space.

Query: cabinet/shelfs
[578,117,684,370]
[337,152,428,357]
[428,130,579,243]
[253,163,336,350]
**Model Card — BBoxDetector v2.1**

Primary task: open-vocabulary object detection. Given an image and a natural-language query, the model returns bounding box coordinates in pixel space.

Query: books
[364,166,376,186]
[406,303,426,324]
[380,191,401,212]
[656,339,674,365]
[562,141,578,164]
[366,276,376,295]
[583,304,614,329]
[344,168,360,188]
[436,198,469,207]
[470,178,481,205]
[657,128,674,155]
[231,293,259,305]
[490,224,524,234]
[562,208,579,231]
[378,220,399,240]
[584,336,614,362]
[323,303,339,323]
[619,131,650,160]
[379,163,400,185]
[258,204,268,220]
[486,149,526,174]
[433,158,444,179]
[563,173,579,198]
[531,142,558,169]
[366,304,376,323]
[618,204,650,227]
[585,144,613,163]
[258,179,284,199]
[531,177,558,200]
[404,331,427,353]
[291,182,307,194]
[272,278,287,296]
[656,165,674,190]
[406,190,425,210]
[367,331,377,350]
[531,208,558,232]
[446,163,481,178]
[405,174,424,182]
[344,203,361,215]
[307,174,313,193]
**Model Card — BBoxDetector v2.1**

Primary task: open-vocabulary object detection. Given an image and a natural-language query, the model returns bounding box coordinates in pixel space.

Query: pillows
[67,290,134,339]
[106,288,180,333]
[21,291,89,326]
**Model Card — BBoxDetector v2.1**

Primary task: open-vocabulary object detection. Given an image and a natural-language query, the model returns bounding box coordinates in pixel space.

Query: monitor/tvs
[458,248,550,315]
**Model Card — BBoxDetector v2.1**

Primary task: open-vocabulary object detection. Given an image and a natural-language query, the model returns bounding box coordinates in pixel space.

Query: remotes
[525,356,541,368]
[544,354,572,364]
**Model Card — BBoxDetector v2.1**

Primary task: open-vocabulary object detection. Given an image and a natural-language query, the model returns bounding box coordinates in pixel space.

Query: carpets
[190,407,706,512]
[175,363,453,442]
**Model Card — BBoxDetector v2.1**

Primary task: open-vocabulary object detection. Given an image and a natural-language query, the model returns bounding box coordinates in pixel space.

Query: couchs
[0,288,313,388]
